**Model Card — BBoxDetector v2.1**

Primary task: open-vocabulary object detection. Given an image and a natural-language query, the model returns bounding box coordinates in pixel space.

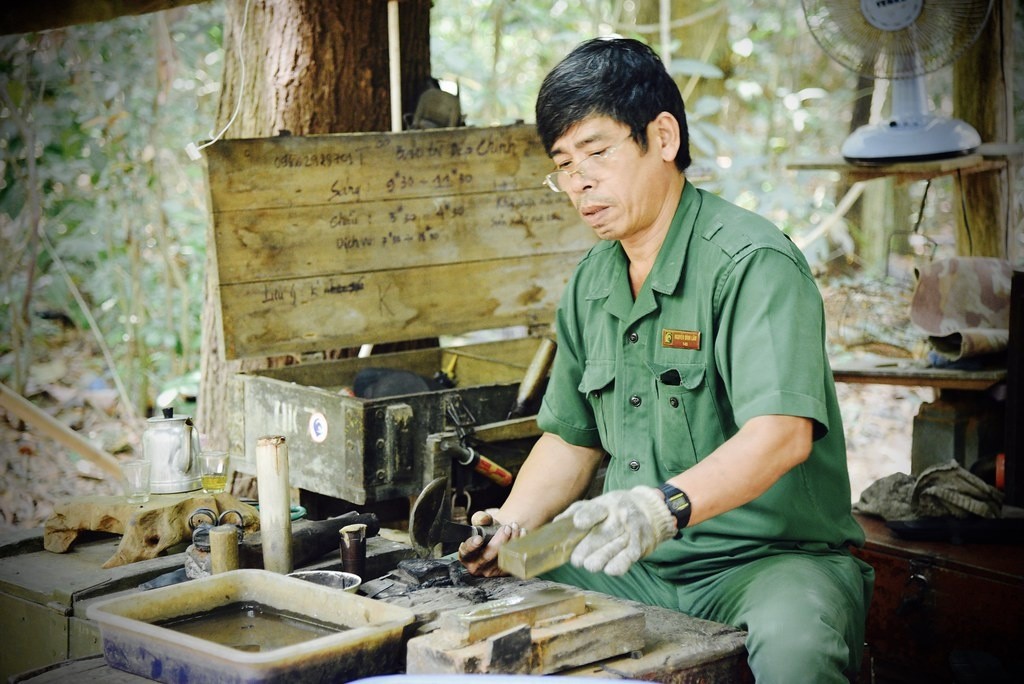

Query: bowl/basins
[286,570,362,594]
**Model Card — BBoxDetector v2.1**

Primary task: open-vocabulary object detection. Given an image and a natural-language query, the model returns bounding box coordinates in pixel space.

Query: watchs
[656,484,692,539]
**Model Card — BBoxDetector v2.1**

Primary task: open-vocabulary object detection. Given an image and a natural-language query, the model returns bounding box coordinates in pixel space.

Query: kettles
[144,407,203,494]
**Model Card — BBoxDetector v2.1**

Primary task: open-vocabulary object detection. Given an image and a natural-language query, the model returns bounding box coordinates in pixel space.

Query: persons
[458,38,874,684]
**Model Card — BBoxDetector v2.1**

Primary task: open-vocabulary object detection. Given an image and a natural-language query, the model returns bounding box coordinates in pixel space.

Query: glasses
[542,134,632,192]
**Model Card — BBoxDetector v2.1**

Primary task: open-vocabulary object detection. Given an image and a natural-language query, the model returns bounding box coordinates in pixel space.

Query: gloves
[552,484,678,575]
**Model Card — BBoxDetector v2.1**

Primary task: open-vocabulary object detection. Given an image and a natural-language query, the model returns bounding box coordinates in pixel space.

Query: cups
[198,451,231,493]
[119,460,151,503]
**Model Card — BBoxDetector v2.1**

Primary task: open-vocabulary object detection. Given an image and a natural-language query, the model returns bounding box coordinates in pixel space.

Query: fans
[799,0,994,165]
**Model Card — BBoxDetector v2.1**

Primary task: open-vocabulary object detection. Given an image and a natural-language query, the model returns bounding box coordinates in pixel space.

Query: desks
[15,577,750,684]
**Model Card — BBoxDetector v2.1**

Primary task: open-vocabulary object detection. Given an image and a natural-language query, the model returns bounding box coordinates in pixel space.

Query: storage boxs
[85,568,416,684]
[852,505,1024,684]
[240,331,560,505]
[0,540,153,684]
